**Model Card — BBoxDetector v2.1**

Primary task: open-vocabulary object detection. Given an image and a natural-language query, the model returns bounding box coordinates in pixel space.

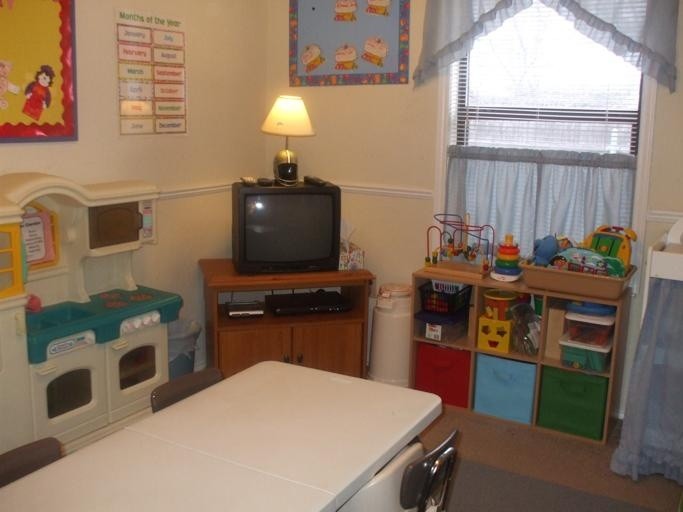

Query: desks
[0,357,449,510]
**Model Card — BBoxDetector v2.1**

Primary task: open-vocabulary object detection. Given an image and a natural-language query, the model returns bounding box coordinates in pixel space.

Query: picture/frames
[288,1,411,86]
[0,1,78,142]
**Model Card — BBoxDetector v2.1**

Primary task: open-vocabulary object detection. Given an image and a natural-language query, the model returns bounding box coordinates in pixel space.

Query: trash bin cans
[166,318,202,382]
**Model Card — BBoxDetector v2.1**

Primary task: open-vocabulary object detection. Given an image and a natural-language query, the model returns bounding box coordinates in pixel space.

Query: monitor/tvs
[232,182,341,273]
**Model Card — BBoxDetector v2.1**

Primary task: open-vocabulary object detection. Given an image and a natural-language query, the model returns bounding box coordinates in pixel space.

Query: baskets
[419,279,471,314]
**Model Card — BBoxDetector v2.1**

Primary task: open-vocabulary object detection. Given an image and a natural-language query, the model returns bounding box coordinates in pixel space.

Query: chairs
[151,366,221,411]
[0,436,63,497]
[354,433,456,507]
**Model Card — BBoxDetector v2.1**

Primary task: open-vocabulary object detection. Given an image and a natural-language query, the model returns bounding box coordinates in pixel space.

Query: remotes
[259,178,272,186]
[304,175,325,187]
[240,176,256,185]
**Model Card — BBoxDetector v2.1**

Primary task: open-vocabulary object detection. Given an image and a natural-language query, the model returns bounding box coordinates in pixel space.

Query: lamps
[257,94,315,186]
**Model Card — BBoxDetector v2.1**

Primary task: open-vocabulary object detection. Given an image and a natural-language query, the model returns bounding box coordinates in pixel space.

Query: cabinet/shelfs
[0,301,34,454]
[107,321,168,430]
[197,256,370,378]
[34,343,106,451]
[408,258,631,447]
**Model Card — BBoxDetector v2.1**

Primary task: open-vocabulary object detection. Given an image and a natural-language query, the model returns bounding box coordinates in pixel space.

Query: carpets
[434,453,654,512]
